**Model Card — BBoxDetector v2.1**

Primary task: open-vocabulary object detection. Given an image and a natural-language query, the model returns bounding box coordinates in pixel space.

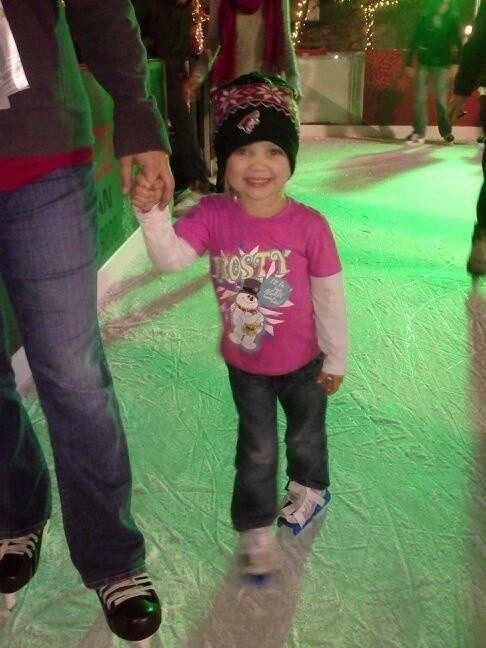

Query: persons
[448,1,486,277]
[135,3,220,195]
[0,0,178,642]
[132,72,348,577]
[397,1,461,145]
[184,1,299,100]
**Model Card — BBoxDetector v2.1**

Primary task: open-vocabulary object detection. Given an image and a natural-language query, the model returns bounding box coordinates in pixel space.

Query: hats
[204,71,300,174]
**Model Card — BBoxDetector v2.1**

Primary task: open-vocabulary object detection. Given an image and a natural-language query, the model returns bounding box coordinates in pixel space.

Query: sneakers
[0,523,45,594]
[468,222,486,275]
[408,133,424,140]
[278,478,331,535]
[95,570,161,641]
[240,528,277,583]
[444,132,454,142]
